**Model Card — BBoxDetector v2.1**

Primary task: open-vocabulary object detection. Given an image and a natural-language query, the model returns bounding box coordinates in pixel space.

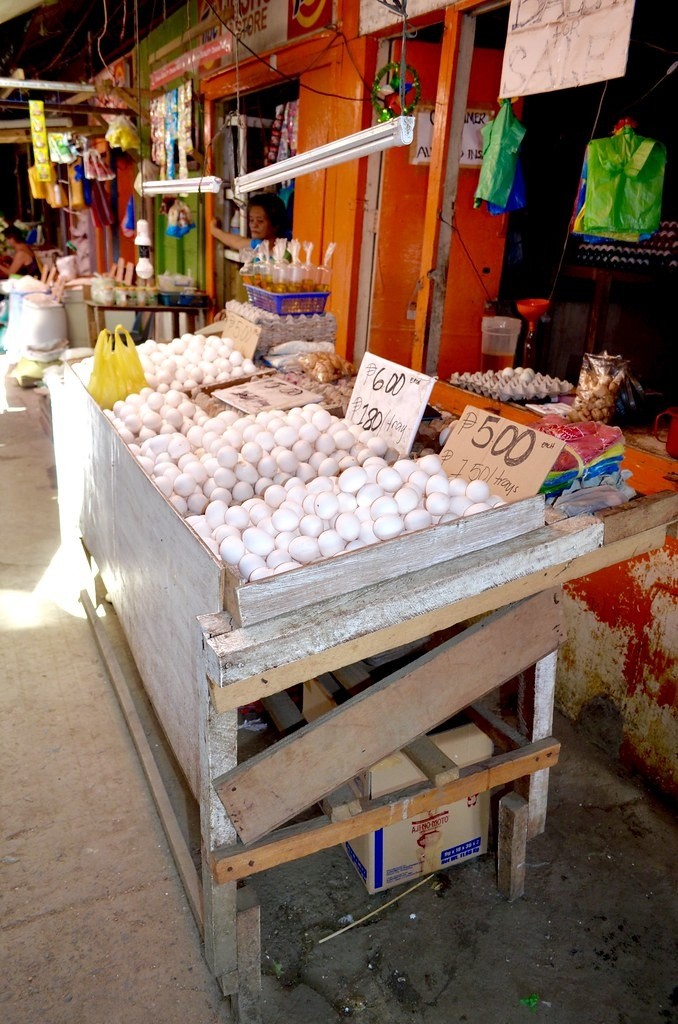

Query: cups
[481,316,521,374]
[655,408,678,460]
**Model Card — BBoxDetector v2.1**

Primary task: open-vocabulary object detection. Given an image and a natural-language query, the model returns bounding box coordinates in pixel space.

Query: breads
[568,371,627,428]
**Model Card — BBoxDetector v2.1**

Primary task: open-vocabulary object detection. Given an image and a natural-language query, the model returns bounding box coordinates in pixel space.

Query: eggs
[579,220,678,268]
[70,333,535,583]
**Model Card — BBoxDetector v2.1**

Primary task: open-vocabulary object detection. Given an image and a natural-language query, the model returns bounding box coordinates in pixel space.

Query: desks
[86,298,209,346]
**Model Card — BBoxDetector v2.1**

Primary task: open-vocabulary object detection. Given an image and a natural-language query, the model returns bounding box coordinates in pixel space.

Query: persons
[211,192,291,262]
[0,227,41,281]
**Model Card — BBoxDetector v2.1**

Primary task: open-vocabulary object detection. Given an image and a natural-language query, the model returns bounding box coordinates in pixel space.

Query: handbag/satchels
[86,325,149,410]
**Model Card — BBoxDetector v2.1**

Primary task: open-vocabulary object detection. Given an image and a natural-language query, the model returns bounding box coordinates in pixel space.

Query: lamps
[124,0,415,196]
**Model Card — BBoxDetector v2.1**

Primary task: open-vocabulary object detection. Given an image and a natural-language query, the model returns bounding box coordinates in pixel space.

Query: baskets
[242,281,331,317]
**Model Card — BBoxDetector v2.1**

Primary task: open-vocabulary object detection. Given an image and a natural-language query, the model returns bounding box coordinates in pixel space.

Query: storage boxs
[300,680,492,896]
[61,340,548,629]
